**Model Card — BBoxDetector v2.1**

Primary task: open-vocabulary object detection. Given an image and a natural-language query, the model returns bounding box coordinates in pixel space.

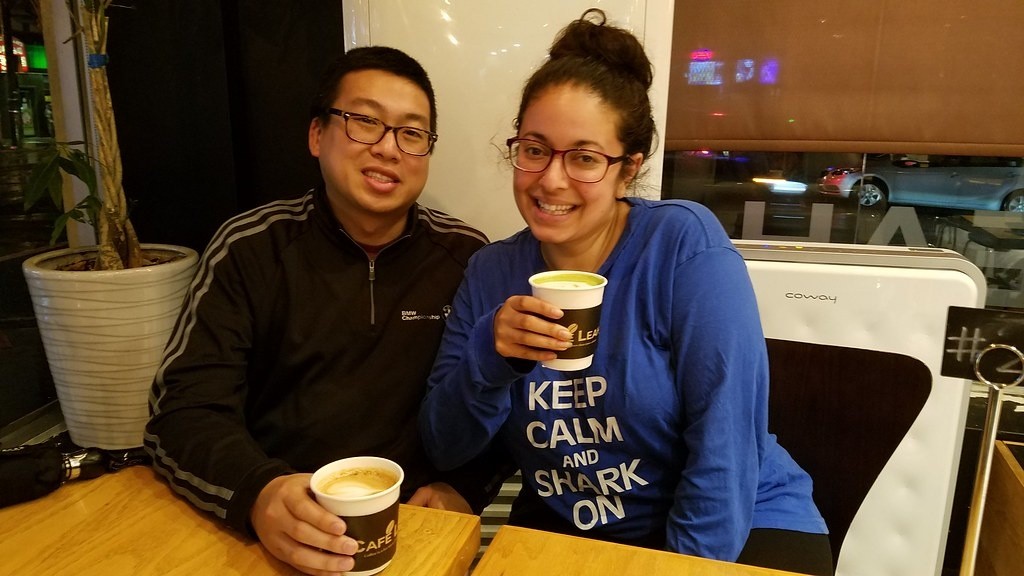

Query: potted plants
[19,1,198,451]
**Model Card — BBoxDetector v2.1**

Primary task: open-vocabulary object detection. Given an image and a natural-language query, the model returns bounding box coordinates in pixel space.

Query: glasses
[327,106,438,156]
[506,135,630,183]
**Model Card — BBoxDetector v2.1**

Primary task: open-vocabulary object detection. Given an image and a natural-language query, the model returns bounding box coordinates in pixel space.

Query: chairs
[732,237,987,575]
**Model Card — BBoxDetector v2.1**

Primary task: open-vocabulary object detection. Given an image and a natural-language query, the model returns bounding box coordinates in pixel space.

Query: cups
[528,270,608,371]
[310,456,404,575]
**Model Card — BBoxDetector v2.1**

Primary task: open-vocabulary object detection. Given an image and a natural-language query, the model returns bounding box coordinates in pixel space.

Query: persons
[140,44,492,576]
[419,6,837,574]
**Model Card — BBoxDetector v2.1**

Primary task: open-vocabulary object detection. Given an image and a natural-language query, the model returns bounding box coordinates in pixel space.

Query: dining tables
[466,524,814,575]
[1,458,482,576]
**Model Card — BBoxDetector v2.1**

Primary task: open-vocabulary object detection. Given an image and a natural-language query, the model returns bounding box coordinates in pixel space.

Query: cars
[816,152,1024,215]
